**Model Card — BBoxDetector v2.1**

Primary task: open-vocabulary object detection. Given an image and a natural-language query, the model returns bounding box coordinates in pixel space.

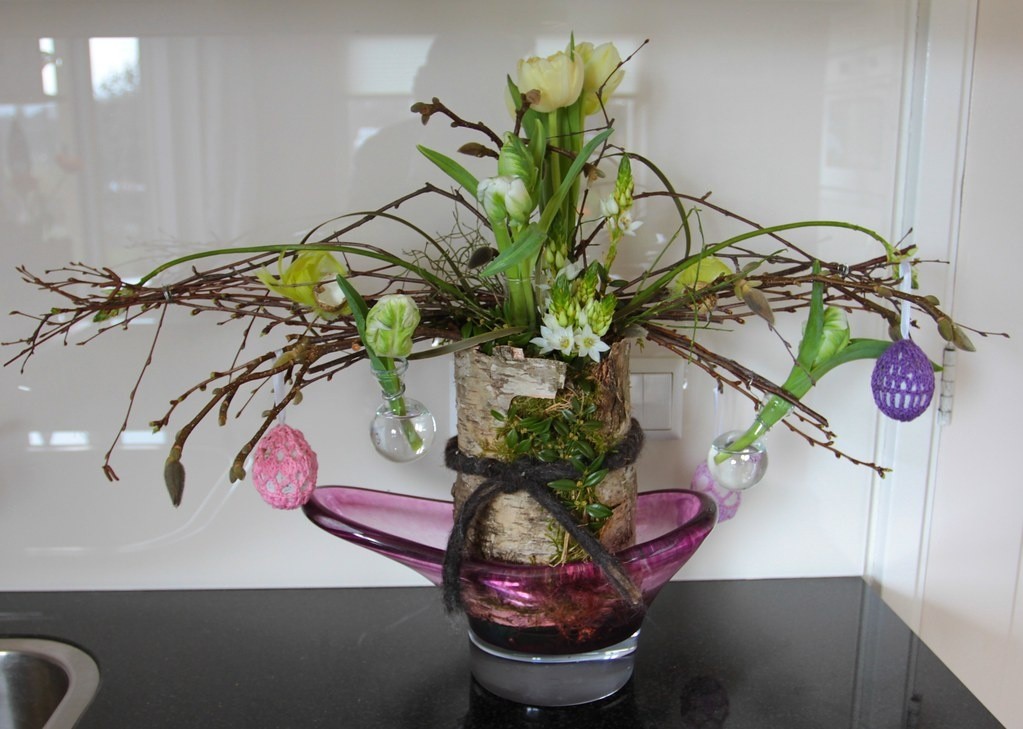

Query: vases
[290,474,723,709]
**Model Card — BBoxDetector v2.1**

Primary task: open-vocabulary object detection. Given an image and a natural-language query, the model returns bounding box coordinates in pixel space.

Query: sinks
[0,633,101,729]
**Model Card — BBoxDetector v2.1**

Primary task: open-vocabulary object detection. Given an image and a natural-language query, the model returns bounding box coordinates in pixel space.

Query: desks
[2,565,1008,729]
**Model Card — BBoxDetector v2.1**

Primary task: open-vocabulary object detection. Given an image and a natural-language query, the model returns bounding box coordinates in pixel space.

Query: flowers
[2,36,1017,567]
